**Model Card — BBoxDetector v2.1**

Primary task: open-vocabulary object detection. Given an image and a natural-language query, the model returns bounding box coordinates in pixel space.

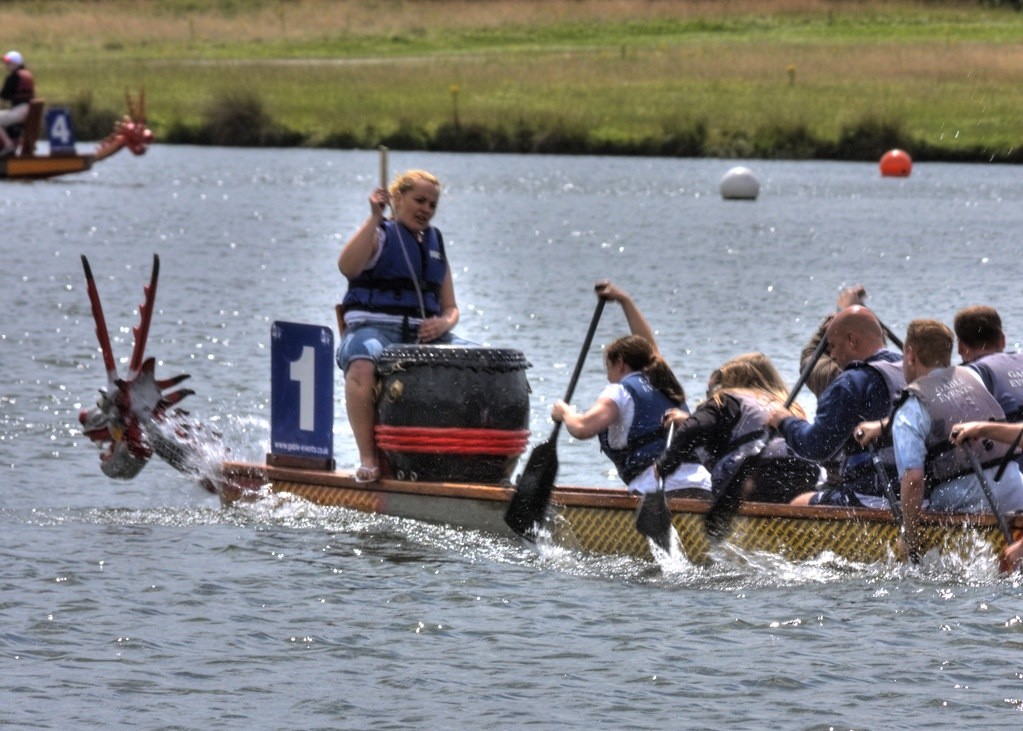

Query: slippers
[354,465,381,483]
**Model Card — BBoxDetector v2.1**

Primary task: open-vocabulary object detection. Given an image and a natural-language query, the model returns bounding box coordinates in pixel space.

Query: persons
[853,321,1023,555]
[800,289,859,398]
[769,305,931,510]
[651,354,821,501]
[953,306,1023,422]
[949,422,1023,564]
[0,51,34,156]
[339,172,481,484]
[551,283,712,498]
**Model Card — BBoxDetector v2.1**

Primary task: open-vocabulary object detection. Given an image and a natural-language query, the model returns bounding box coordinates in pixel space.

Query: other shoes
[0,145,15,159]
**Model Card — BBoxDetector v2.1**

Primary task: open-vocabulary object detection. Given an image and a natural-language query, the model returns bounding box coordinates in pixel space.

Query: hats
[2,51,23,65]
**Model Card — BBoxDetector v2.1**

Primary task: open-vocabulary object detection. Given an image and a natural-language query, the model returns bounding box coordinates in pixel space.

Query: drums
[368,342,531,485]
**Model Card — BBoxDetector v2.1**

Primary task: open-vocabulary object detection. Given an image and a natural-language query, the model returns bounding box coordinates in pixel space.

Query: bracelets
[880,417,884,432]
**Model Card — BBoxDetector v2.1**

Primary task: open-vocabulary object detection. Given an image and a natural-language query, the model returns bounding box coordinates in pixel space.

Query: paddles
[863,294,906,355]
[636,422,678,542]
[957,426,1011,544]
[505,298,608,540]
[704,315,839,540]
[863,422,922,573]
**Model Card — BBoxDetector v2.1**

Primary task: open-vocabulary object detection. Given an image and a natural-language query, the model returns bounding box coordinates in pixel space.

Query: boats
[74,253,1023,571]
[1,89,152,178]
